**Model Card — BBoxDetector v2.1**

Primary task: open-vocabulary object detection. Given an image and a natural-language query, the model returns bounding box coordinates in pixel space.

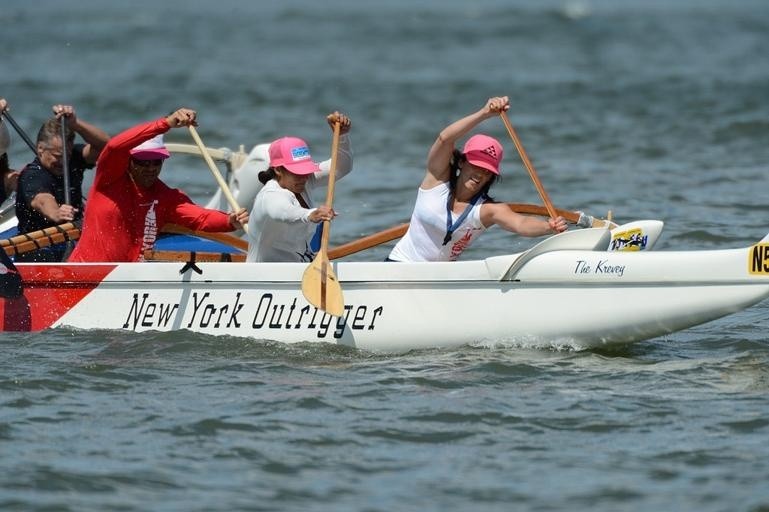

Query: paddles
[303,113,344,317]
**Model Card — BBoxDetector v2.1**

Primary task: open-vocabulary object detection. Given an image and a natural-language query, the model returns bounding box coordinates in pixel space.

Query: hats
[463,134,505,176]
[268,136,322,175]
[129,135,171,158]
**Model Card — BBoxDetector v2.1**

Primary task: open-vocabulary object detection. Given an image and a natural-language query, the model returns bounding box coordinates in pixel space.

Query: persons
[382,95,571,263]
[244,109,355,262]
[14,103,111,263]
[0,98,22,207]
[66,107,250,263]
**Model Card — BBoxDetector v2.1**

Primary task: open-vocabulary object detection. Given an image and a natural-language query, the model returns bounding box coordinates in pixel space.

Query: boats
[0,186,769,355]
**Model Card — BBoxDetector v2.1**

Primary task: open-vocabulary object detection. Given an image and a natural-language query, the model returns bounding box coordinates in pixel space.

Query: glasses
[134,156,162,168]
